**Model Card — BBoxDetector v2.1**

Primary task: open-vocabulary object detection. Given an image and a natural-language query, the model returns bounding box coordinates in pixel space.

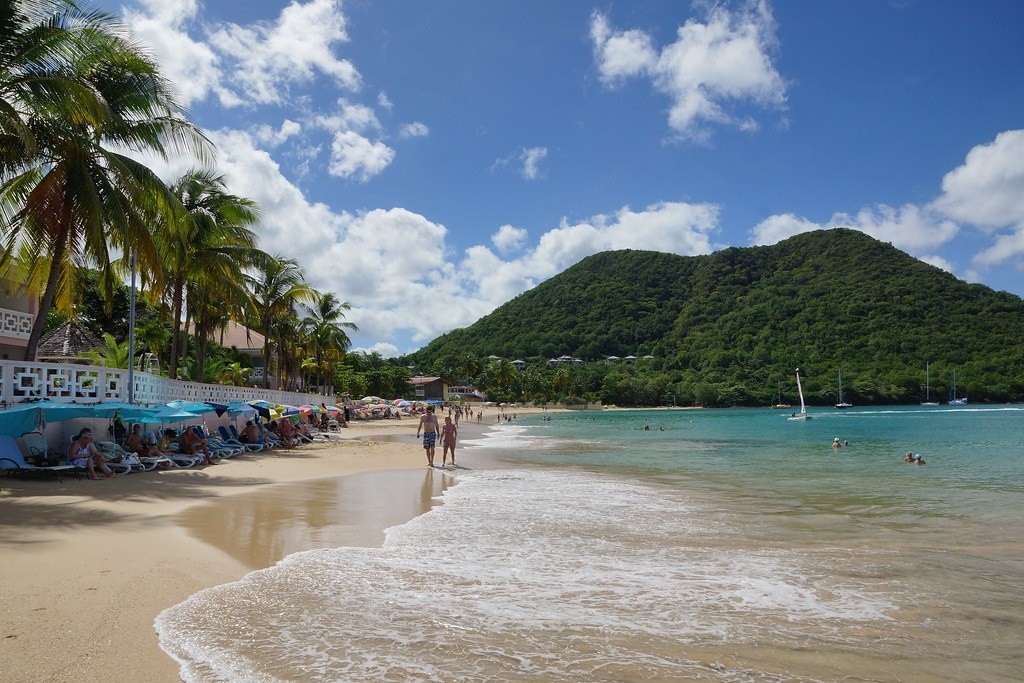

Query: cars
[0,373,18,393]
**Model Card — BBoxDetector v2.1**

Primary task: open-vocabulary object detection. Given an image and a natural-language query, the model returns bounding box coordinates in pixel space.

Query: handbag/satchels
[30,446,48,466]
[47,447,60,466]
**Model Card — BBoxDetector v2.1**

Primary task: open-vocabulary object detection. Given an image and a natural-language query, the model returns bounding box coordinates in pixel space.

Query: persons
[904,452,916,462]
[496,405,517,423]
[383,401,483,427]
[240,420,272,451]
[125,423,159,457]
[832,438,841,448]
[844,440,848,446]
[439,416,457,466]
[272,403,350,449]
[914,454,926,465]
[417,406,439,467]
[155,426,219,465]
[65,428,122,480]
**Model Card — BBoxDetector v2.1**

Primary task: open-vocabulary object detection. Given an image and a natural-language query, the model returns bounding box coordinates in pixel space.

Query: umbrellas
[224,399,344,428]
[0,396,228,460]
[342,396,430,417]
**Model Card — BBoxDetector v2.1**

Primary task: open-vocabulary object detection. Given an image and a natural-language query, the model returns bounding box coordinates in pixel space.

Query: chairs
[0,423,315,483]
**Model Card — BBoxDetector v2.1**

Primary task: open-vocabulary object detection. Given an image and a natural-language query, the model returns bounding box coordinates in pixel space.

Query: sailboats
[769,378,791,409]
[921,361,939,406]
[949,369,967,406]
[787,367,812,421]
[835,369,853,408]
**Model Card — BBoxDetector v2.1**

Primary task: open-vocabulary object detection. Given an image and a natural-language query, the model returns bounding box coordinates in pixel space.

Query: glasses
[82,432,91,438]
